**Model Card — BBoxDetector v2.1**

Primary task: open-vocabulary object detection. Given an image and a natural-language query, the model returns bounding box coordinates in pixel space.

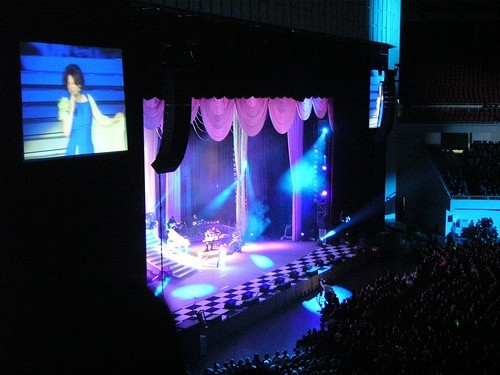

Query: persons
[146,212,500,375]
[57,63,125,157]
[373,85,385,128]
